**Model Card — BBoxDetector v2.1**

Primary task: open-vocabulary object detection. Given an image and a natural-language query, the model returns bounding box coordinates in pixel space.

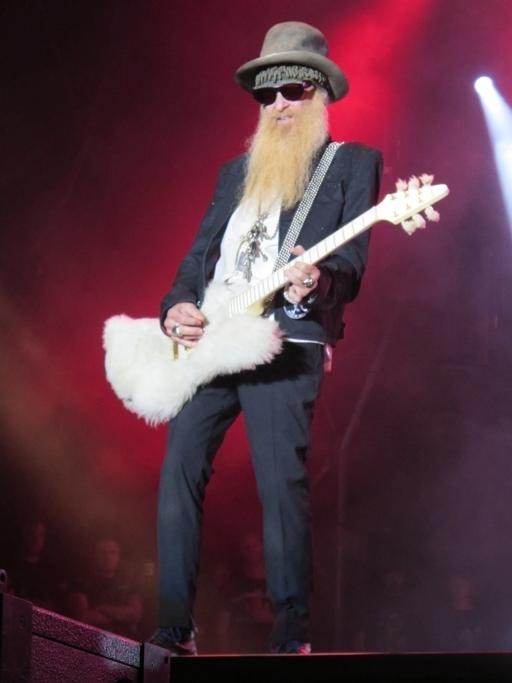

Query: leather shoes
[272,641,311,653]
[144,627,198,655]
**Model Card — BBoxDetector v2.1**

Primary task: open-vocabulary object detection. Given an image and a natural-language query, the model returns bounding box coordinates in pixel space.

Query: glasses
[250,83,312,105]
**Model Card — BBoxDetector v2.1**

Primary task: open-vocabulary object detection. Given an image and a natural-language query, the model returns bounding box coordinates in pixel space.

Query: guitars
[102,172,450,427]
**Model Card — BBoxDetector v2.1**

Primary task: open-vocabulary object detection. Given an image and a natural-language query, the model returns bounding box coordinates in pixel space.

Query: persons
[74,539,145,639]
[149,19,382,655]
[4,516,67,611]
[428,568,495,653]
[213,534,281,651]
[351,568,423,652]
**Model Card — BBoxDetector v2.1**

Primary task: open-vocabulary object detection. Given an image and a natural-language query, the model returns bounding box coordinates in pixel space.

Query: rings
[170,324,182,337]
[304,277,314,287]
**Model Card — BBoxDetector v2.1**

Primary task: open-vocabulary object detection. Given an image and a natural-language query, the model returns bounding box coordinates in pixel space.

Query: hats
[234,21,349,102]
